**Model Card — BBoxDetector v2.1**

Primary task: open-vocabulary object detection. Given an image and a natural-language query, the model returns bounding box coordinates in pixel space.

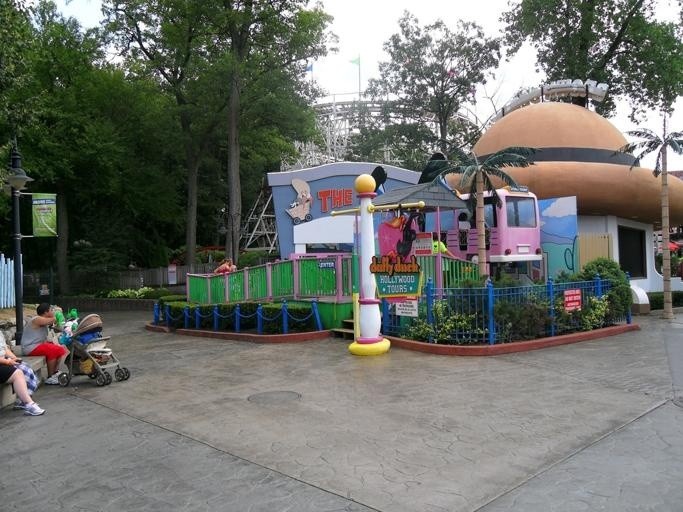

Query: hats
[63,320,72,327]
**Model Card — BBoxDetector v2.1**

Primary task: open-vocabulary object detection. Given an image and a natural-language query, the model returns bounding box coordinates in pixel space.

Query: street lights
[5,133,35,343]
[217,210,235,266]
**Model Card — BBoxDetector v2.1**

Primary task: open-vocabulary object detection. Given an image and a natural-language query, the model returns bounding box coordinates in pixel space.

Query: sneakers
[23,402,45,416]
[12,398,26,409]
[43,370,62,385]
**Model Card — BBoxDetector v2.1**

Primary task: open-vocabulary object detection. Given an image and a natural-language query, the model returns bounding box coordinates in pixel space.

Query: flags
[31,192,58,237]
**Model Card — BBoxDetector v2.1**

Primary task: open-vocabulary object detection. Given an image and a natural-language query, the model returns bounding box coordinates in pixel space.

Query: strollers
[47,313,130,386]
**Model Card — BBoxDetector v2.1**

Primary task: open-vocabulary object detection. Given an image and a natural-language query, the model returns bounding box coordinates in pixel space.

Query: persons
[433,233,460,289]
[228,257,238,272]
[457,213,471,230]
[21,300,65,386]
[470,210,489,229]
[213,256,233,275]
[0,330,46,417]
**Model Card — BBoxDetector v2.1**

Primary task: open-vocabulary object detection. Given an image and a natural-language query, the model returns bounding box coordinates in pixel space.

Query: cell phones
[15,358,23,363]
